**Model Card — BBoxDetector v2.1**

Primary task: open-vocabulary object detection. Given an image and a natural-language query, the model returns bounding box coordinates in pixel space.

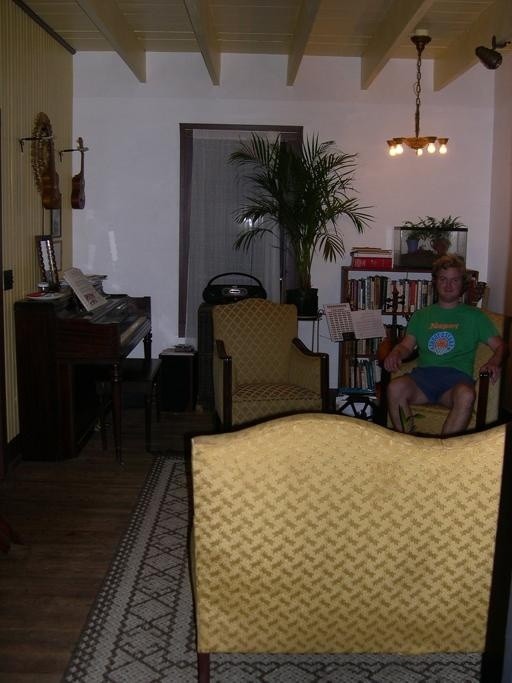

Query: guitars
[71,137,86,209]
[42,127,61,210]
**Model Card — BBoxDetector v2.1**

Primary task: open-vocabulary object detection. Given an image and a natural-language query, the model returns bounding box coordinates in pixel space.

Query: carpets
[60,454,481,683]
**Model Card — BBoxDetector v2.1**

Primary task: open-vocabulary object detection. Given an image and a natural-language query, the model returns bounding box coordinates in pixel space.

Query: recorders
[201,271,268,306]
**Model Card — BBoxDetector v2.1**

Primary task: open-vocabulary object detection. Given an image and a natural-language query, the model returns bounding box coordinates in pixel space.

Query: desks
[337,390,382,425]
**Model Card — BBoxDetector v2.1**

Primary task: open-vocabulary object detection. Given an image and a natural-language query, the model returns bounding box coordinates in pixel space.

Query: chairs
[190,412,510,683]
[377,311,505,438]
[197,295,331,432]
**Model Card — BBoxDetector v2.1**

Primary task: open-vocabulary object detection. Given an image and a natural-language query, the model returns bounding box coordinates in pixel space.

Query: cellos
[372,287,415,426]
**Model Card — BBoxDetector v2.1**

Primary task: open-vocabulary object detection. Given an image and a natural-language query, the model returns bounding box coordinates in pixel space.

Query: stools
[96,359,162,452]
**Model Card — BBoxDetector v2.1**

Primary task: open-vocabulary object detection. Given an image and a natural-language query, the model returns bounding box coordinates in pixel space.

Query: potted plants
[227,130,375,315]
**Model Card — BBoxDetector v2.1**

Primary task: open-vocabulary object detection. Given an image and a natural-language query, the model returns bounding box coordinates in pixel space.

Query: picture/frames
[54,240,62,271]
[44,208,62,238]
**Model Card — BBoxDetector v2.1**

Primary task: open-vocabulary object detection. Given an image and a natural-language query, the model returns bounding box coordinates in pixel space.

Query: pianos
[14,276,151,461]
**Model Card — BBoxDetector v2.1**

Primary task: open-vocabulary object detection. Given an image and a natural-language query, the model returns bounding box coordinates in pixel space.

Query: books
[349,251,392,258]
[355,249,393,252]
[349,275,439,398]
[323,301,356,343]
[63,266,108,312]
[351,246,381,250]
[351,257,393,268]
[84,273,107,282]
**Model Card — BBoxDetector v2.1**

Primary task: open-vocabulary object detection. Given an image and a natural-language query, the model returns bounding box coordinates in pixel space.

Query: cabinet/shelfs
[338,267,478,399]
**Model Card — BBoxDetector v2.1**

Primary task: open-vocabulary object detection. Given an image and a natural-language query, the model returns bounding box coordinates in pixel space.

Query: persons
[383,252,506,436]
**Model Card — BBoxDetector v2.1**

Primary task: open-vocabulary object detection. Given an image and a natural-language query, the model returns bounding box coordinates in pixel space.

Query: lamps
[476,35,512,70]
[387,29,449,158]
